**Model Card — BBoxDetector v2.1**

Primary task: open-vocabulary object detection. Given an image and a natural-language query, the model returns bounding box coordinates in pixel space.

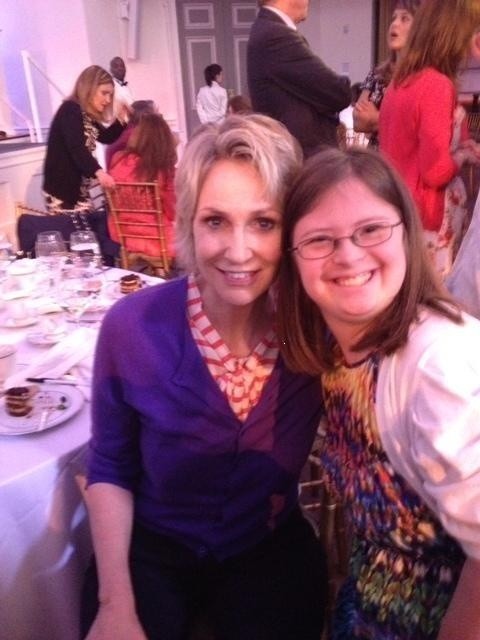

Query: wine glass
[34,231,103,293]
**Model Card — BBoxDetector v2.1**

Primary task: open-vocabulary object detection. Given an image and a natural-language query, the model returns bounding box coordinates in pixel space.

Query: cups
[39,306,66,339]
[7,258,49,292]
[3,290,34,321]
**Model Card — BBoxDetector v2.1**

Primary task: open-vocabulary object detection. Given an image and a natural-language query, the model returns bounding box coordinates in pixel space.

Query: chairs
[103,178,175,279]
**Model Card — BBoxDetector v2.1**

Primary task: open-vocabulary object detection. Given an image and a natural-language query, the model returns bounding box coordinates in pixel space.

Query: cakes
[4,386,29,415]
[120,273,140,294]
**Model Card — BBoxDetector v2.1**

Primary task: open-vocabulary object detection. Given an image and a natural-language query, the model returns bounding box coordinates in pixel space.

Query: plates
[1,381,85,435]
[27,332,64,346]
[0,315,39,329]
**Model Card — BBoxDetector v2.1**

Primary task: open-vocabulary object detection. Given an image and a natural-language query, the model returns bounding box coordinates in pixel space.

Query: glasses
[290,220,403,260]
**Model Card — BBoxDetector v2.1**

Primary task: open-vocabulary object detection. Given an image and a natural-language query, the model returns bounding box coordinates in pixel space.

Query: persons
[247,1,350,156]
[42,57,180,270]
[273,147,480,640]
[351,0,479,273]
[76,111,334,640]
[195,65,227,124]
